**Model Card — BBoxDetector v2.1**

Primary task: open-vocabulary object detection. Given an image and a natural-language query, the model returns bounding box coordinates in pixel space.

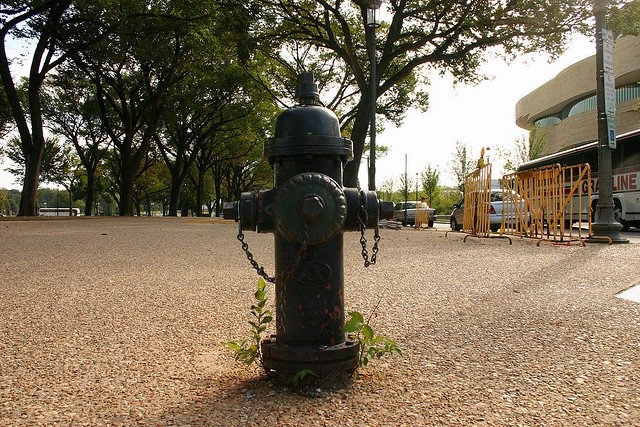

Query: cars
[450,189,531,232]
[393,201,437,227]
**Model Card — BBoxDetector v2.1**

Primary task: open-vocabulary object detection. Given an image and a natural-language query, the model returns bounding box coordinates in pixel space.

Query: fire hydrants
[223,72,393,397]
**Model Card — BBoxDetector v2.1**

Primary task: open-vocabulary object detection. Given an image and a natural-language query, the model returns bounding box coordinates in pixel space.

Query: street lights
[353,1,382,190]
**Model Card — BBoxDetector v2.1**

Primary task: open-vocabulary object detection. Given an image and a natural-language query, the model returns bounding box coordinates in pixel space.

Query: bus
[516,129,640,232]
[39,208,80,216]
